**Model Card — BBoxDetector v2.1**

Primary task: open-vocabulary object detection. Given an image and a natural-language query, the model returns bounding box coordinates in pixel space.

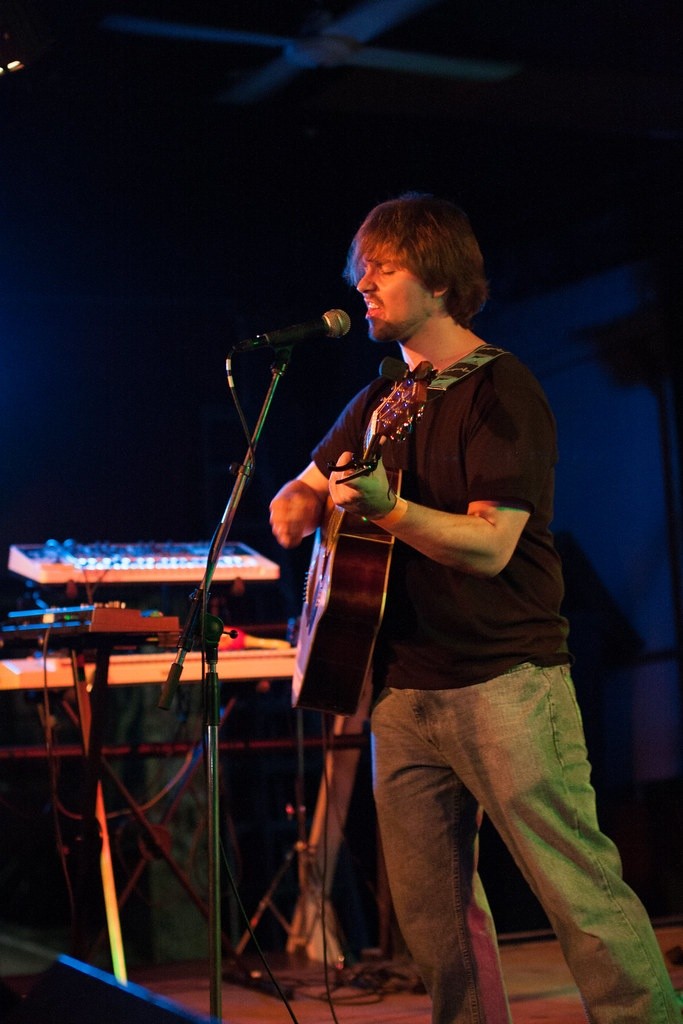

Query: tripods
[226,710,355,973]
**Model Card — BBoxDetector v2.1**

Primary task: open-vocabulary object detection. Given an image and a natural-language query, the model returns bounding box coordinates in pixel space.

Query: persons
[268,197,683,1024]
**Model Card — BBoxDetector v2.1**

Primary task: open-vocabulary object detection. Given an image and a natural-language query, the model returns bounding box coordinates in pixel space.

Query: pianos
[0,539,284,587]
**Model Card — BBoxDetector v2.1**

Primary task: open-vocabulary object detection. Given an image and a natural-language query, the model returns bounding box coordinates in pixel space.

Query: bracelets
[370,495,408,531]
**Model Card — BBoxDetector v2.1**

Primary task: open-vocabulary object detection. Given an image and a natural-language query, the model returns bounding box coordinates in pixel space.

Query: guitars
[290,353,433,720]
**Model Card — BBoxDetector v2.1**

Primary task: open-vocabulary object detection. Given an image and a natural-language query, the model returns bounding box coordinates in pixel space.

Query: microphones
[233,309,351,352]
[379,357,415,381]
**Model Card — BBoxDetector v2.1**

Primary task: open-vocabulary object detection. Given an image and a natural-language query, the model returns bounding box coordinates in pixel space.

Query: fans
[97,0,527,116]
